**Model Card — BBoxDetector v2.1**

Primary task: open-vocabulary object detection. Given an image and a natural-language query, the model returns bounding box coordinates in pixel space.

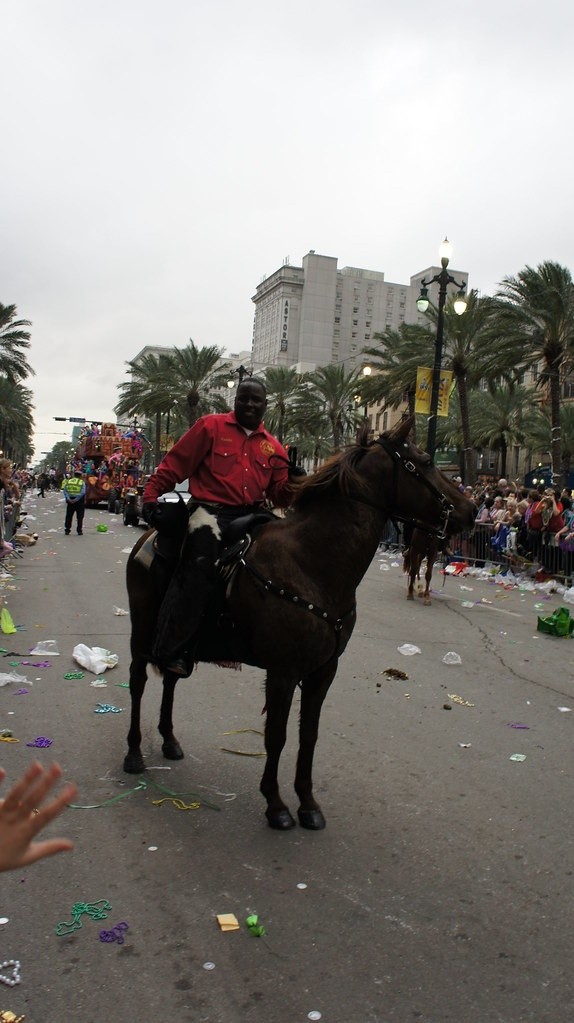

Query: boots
[167,555,209,676]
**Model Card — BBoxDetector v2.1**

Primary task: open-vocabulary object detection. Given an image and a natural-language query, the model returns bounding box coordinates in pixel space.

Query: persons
[0,425,152,557]
[142,378,307,677]
[380,475,574,578]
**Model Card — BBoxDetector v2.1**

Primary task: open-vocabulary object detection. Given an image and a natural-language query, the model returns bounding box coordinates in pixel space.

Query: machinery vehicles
[108,476,143,514]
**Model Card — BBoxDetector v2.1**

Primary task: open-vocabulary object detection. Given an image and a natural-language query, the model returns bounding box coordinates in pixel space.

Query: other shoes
[0,545,13,558]
[65,531,70,535]
[2,541,13,547]
[78,531,83,535]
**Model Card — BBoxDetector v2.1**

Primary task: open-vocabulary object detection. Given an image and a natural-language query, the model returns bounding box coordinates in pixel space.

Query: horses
[121,416,479,831]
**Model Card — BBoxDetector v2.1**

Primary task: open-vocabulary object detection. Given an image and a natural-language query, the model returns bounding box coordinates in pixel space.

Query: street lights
[127,417,142,466]
[364,366,372,415]
[227,365,252,389]
[533,463,545,491]
[66,448,76,476]
[415,258,469,464]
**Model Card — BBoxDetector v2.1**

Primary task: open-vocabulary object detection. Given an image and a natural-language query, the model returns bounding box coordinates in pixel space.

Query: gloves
[287,466,307,485]
[142,503,160,524]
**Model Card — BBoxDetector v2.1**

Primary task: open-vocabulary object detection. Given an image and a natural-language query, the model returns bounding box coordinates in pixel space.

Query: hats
[75,471,83,474]
[453,477,462,482]
[519,500,529,509]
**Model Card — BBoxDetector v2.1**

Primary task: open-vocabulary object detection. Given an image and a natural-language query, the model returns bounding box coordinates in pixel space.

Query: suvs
[122,474,191,531]
